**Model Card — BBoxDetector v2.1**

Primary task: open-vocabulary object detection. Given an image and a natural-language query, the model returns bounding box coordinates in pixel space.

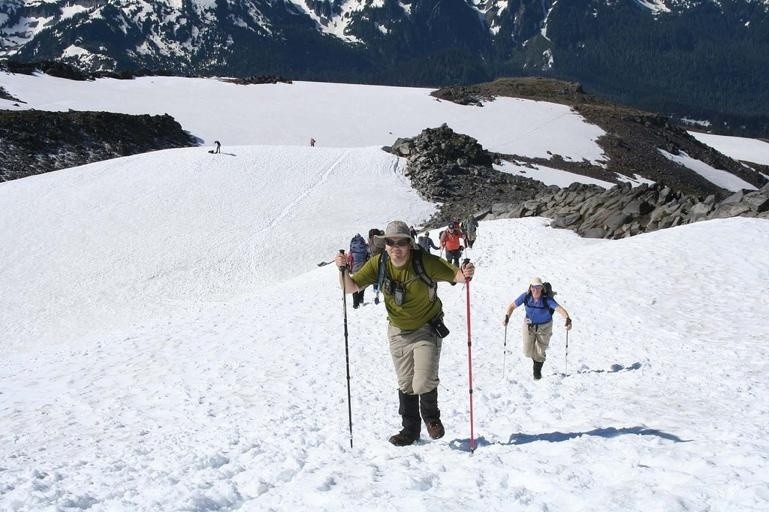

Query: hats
[528,277,544,286]
[425,222,454,235]
[374,220,415,249]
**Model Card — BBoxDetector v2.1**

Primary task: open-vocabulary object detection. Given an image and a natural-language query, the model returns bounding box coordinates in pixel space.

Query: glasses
[384,238,411,247]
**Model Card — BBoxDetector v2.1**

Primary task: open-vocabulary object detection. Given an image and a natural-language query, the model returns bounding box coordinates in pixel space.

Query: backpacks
[524,282,554,316]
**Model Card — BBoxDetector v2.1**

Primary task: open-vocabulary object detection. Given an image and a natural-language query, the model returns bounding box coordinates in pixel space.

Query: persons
[461,216,470,248]
[440,221,465,285]
[214,141,221,154]
[335,220,475,447]
[367,239,385,305]
[348,244,365,310]
[419,231,440,254]
[465,215,479,248]
[311,138,316,147]
[409,226,417,240]
[500,276,573,381]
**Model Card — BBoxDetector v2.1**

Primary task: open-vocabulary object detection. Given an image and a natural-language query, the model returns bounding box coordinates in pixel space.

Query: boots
[420,386,445,440]
[389,388,421,447]
[533,358,544,381]
